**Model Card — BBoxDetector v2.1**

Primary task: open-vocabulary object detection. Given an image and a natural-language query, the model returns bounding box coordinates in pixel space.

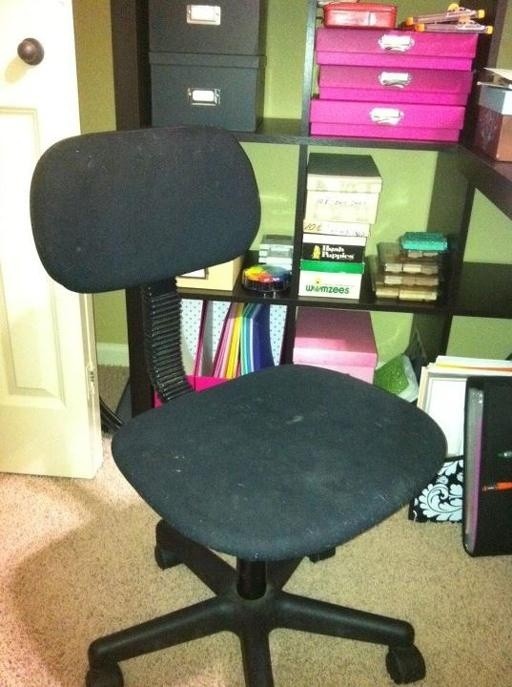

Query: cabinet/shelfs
[110,0,512,419]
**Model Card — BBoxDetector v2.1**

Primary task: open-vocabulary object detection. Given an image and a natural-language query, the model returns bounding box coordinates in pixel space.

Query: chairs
[29,124,446,687]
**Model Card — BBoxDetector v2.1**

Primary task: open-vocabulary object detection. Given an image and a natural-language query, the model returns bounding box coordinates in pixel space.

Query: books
[211,301,275,378]
[372,352,512,524]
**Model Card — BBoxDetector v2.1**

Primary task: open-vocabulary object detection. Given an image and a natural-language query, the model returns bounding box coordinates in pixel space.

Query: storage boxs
[175,255,244,292]
[293,307,378,385]
[315,27,479,71]
[310,97,466,142]
[299,259,366,301]
[319,64,473,106]
[305,153,382,224]
[474,85,512,162]
[149,53,266,133]
[149,0,260,55]
[301,221,371,261]
